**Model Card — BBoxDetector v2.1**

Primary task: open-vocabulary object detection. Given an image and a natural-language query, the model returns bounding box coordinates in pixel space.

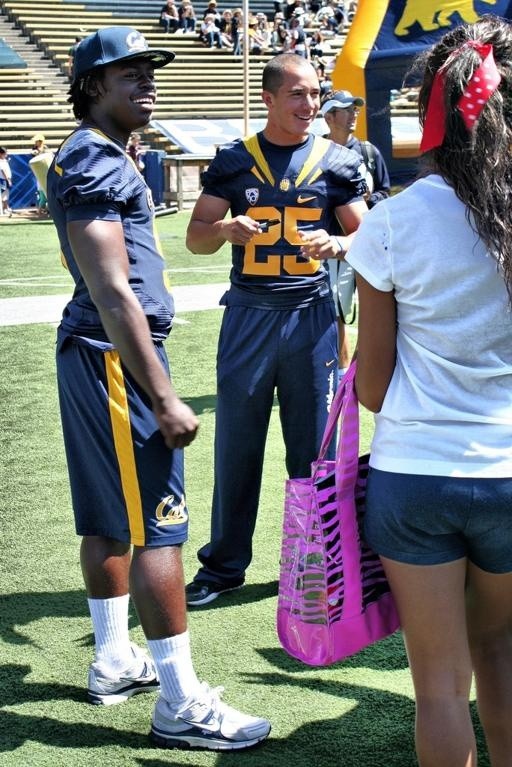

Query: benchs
[0,0,424,155]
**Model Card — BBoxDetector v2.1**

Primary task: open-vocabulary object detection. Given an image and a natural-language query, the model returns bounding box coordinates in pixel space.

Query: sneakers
[185,582,245,606]
[88,643,161,706]
[151,682,271,749]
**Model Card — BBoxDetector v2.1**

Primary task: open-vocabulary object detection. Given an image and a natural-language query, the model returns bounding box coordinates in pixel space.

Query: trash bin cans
[136,150,166,206]
[8,153,36,209]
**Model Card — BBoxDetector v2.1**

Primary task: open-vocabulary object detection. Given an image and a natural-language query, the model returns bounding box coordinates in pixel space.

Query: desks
[160,155,214,210]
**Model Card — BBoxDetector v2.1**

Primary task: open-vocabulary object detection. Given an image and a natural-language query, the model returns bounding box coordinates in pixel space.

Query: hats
[320,91,364,118]
[168,0,304,24]
[32,134,44,142]
[74,28,175,77]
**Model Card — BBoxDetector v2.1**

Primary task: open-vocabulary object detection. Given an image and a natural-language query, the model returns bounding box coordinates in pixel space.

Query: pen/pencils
[256,219,281,228]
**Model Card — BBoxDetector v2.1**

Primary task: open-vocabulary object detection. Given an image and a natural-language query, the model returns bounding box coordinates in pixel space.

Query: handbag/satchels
[278,453,401,666]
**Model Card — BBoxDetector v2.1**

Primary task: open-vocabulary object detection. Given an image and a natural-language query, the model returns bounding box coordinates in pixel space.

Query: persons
[37,179,49,217]
[185,52,371,607]
[127,132,146,172]
[345,18,512,767]
[32,135,51,156]
[46,26,272,751]
[0,146,13,217]
[322,89,390,391]
[159,0,422,102]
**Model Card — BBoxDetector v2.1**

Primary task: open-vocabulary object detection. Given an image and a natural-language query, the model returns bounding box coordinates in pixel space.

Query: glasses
[336,109,360,114]
